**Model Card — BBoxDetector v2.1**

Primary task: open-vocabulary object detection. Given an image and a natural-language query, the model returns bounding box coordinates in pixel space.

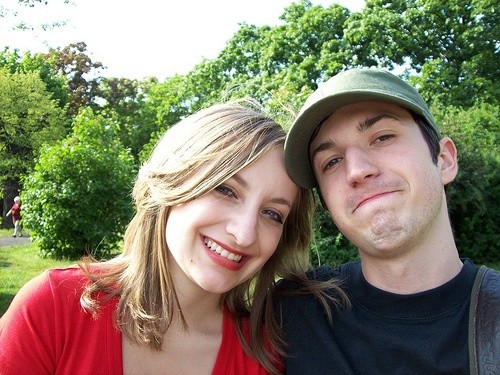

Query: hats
[282,67,442,191]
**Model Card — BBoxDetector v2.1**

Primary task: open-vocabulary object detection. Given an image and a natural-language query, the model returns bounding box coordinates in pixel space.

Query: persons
[0,83,352,375]
[6,196,23,237]
[258,68,500,375]
